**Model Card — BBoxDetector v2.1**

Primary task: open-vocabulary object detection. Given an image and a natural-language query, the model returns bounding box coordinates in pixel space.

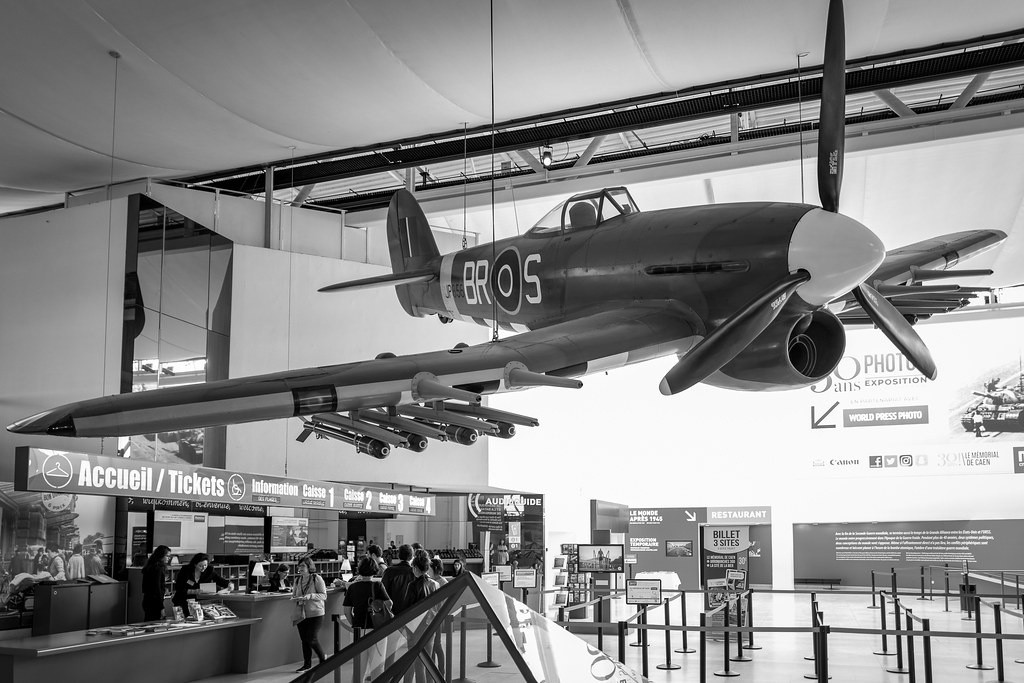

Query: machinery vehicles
[960,366,1024,433]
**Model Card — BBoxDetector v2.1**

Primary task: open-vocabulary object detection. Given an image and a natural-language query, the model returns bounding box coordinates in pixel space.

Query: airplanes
[2,1,1011,460]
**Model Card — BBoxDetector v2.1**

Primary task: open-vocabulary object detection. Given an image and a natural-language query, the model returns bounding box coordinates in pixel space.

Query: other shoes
[296,666,312,673]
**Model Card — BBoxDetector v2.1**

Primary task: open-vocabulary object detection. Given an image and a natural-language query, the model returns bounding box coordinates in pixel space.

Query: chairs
[569,202,597,228]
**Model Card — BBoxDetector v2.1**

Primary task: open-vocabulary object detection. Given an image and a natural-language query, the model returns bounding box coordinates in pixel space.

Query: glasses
[297,565,308,568]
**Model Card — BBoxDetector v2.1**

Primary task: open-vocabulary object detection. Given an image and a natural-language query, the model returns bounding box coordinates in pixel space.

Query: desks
[0,613,264,683]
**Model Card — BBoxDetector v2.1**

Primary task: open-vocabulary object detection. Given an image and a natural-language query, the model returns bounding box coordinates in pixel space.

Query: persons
[451,560,466,577]
[342,540,450,683]
[290,557,327,674]
[266,563,293,592]
[140,545,172,621]
[497,539,508,566]
[489,542,494,567]
[7,539,108,582]
[171,553,234,616]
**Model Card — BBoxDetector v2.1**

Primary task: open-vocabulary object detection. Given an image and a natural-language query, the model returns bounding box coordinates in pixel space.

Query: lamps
[542,147,553,168]
[250,562,266,591]
[341,556,352,581]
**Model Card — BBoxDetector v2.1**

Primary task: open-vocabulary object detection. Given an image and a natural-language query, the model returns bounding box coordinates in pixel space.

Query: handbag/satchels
[369,582,395,629]
[292,603,307,626]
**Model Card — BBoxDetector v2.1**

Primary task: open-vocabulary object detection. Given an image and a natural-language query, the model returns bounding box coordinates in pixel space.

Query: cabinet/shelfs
[32,560,343,635]
